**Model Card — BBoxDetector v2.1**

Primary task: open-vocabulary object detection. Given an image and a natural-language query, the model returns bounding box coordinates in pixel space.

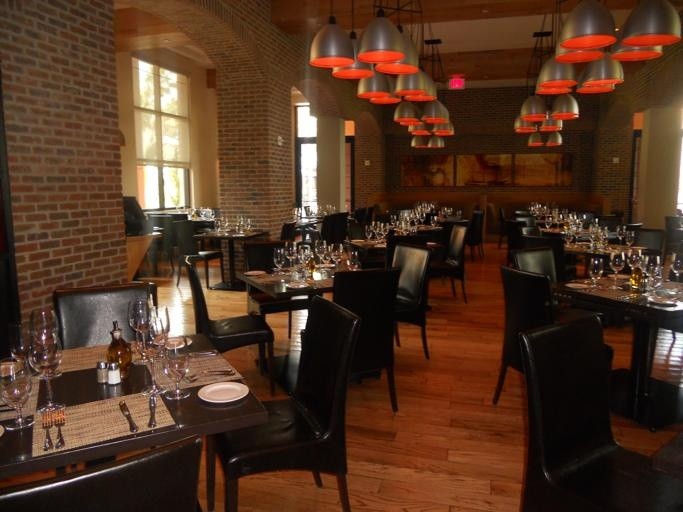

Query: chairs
[219,293,363,510]
[135,205,485,414]
[491,204,683,407]
[50,280,159,350]
[522,315,681,510]
[0,433,204,511]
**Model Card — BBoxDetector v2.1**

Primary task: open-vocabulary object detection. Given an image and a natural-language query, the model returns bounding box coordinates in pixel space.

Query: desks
[0,333,271,512]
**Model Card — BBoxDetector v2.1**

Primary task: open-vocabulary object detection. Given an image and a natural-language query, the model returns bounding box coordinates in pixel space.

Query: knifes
[117,399,139,432]
[147,392,157,429]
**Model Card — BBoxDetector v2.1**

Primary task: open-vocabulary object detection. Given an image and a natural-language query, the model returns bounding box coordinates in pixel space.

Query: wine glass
[135,319,169,395]
[150,302,168,345]
[364,200,462,242]
[159,336,191,401]
[527,202,683,307]
[270,240,358,288]
[126,299,150,368]
[0,308,66,433]
[186,203,334,237]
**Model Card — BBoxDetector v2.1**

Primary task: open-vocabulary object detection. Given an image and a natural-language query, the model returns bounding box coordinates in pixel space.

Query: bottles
[94,361,121,385]
[107,320,132,377]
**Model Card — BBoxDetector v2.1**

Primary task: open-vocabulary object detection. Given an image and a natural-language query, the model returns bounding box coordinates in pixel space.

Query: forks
[181,367,237,385]
[40,408,64,450]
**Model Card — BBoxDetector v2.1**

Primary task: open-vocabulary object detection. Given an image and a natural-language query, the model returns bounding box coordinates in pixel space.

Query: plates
[199,381,248,403]
[164,335,194,349]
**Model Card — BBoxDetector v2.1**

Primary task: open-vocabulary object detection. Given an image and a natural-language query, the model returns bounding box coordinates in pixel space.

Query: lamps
[305,0,456,152]
[504,1,682,150]
